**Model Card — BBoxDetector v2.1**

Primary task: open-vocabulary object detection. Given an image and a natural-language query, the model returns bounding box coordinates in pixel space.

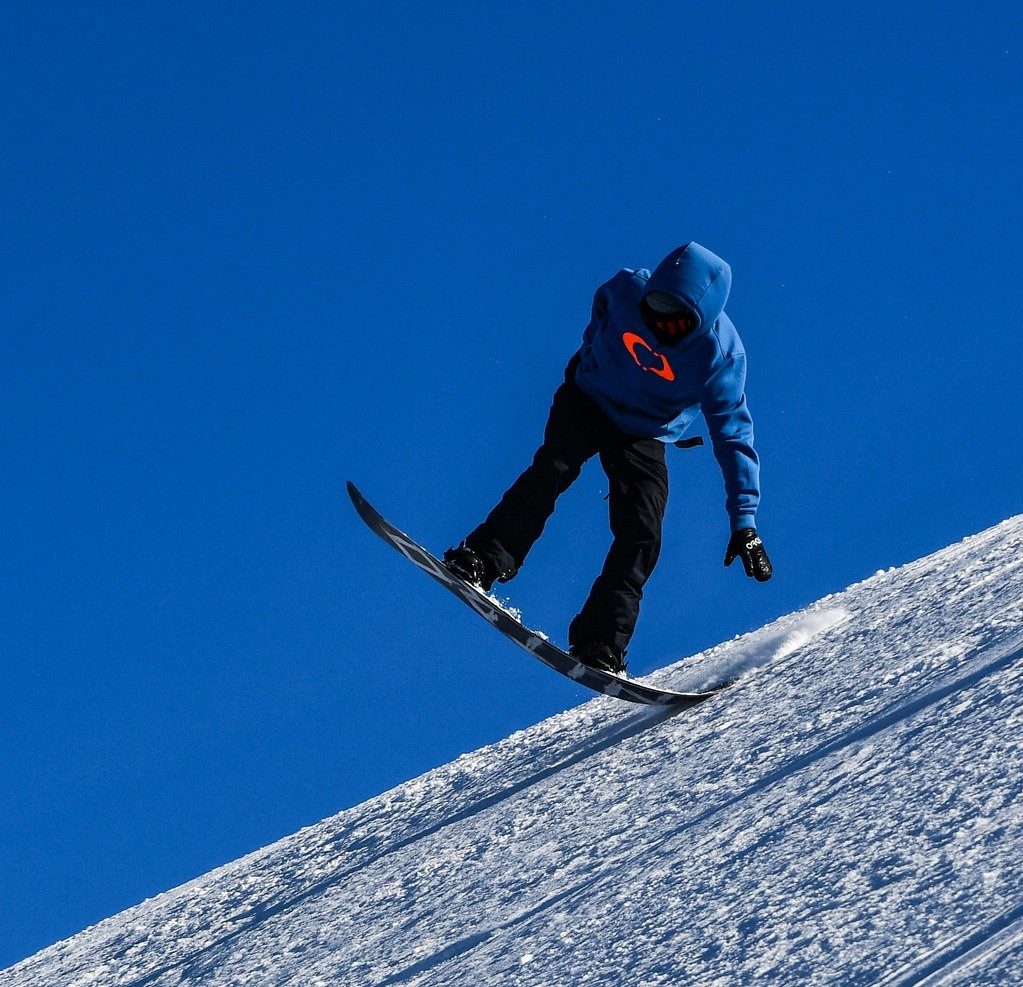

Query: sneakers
[441,538,496,592]
[565,644,628,681]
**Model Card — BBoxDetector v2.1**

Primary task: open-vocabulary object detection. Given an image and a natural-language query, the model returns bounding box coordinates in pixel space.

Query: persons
[449,240,773,678]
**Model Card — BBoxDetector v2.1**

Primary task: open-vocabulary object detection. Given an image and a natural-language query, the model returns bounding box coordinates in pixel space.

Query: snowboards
[343,477,743,704]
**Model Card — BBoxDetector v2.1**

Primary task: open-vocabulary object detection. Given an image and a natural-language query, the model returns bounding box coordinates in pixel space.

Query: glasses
[646,307,696,345]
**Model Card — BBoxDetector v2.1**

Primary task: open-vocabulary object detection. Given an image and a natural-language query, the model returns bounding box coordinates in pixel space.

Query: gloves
[724,528,773,582]
